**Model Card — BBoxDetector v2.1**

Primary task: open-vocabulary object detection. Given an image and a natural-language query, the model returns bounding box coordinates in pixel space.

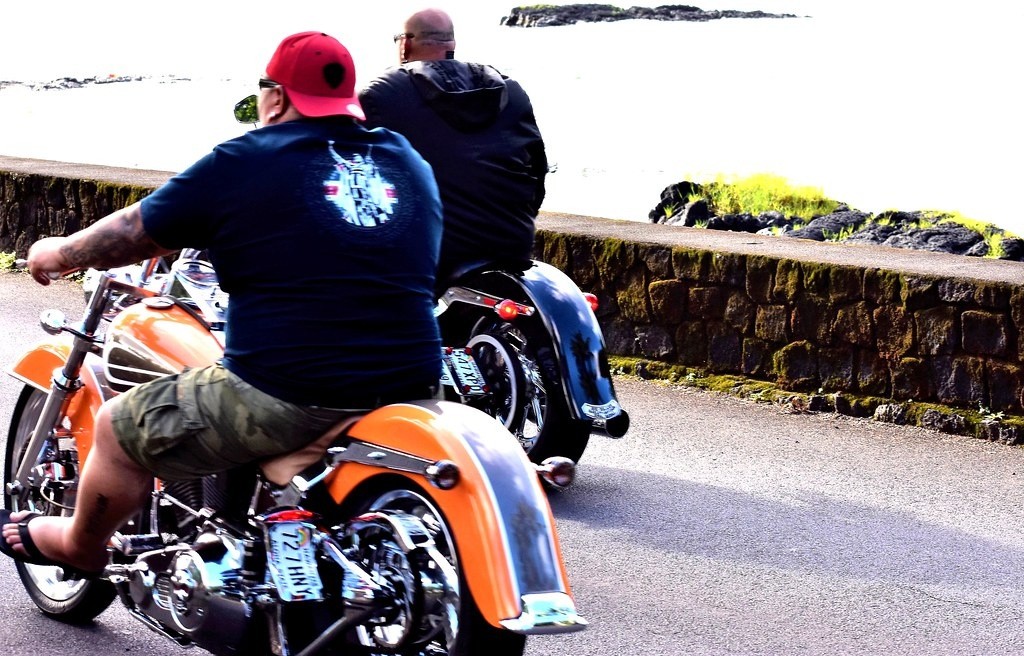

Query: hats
[266,32,366,122]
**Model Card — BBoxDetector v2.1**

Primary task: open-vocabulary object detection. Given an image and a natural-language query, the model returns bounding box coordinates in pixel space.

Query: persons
[1,34,446,574]
[351,7,550,276]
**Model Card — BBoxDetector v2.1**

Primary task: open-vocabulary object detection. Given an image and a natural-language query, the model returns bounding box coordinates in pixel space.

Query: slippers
[0,509,104,579]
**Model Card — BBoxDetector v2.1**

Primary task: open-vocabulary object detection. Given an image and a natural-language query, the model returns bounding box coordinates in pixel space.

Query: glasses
[394,33,413,43]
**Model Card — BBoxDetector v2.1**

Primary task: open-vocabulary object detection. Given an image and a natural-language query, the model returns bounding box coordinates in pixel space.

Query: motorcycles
[86,248,629,495]
[3,258,589,656]
